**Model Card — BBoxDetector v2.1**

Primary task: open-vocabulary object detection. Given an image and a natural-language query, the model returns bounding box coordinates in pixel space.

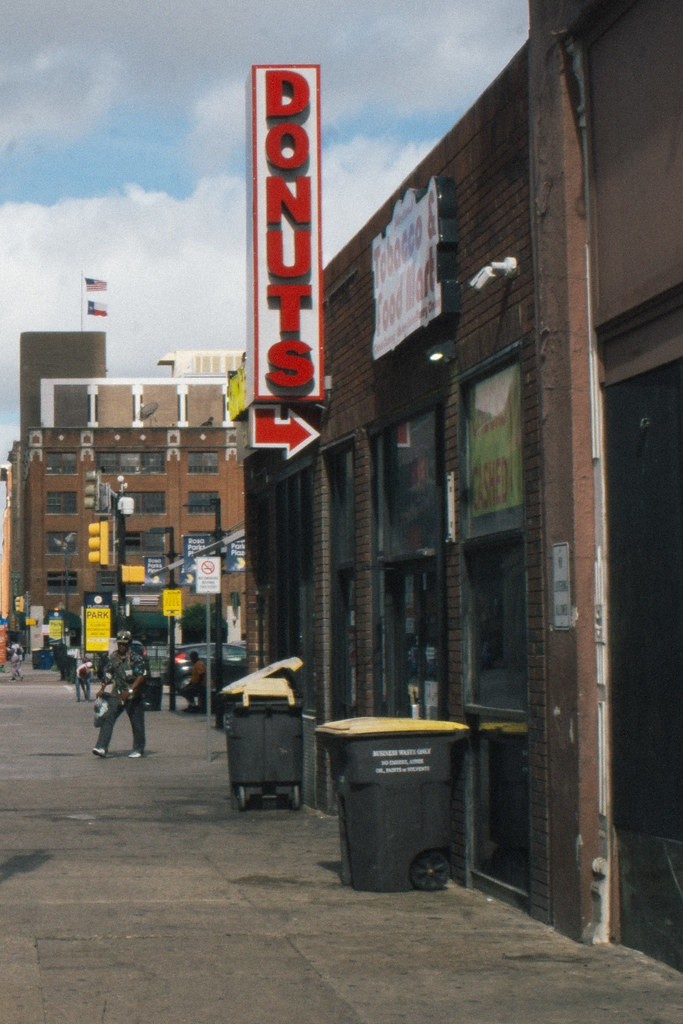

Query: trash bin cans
[144,676,163,711]
[221,694,304,808]
[315,717,469,895]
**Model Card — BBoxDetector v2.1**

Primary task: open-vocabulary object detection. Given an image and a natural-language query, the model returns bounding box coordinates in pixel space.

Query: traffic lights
[87,521,110,565]
[29,618,38,625]
[14,595,24,613]
[83,470,101,511]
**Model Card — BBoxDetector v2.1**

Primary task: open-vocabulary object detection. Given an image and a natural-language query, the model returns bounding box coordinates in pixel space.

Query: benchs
[198,689,216,715]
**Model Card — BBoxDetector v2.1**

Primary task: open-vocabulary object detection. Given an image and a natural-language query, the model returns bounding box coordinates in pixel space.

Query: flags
[87,300,108,317]
[85,277,107,292]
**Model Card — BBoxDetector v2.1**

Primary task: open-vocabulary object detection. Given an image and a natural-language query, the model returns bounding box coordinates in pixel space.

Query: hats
[113,630,132,642]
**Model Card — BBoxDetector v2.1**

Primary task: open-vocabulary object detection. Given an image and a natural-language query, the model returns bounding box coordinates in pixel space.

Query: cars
[161,643,249,694]
[97,640,151,683]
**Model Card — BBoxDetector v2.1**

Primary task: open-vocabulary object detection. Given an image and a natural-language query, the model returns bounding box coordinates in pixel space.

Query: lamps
[470,257,517,290]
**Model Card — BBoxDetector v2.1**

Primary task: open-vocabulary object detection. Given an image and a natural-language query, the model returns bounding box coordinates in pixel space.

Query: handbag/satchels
[93,695,109,727]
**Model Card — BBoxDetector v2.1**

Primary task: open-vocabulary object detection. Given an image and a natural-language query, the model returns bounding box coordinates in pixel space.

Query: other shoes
[92,748,106,757]
[128,752,142,757]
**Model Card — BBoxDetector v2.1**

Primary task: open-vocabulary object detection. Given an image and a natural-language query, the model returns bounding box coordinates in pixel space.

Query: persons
[92,631,150,758]
[180,651,207,712]
[75,662,93,703]
[7,638,23,682]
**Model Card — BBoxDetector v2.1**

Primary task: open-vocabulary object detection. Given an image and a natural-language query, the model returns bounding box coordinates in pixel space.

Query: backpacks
[16,648,21,655]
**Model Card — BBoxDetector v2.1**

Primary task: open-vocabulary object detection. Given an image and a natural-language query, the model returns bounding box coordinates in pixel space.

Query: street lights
[53,532,76,649]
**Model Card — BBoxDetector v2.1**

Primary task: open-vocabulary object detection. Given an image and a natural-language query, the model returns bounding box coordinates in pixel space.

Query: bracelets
[128,689,133,694]
